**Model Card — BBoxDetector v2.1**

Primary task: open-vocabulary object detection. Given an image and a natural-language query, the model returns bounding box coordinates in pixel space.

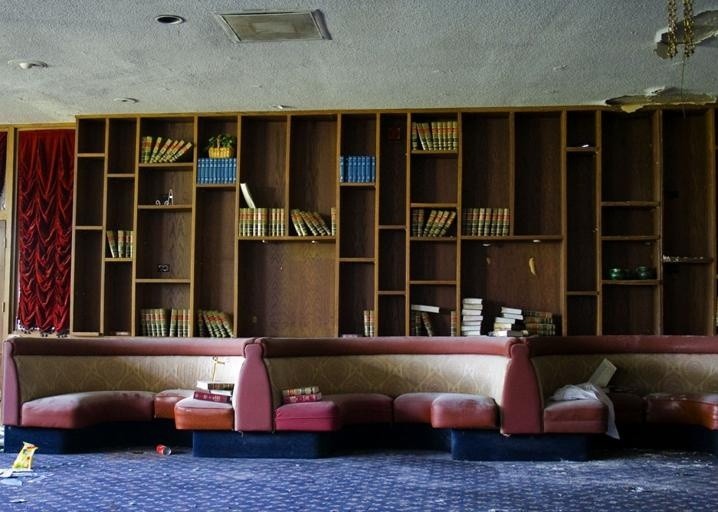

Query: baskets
[209,135,234,158]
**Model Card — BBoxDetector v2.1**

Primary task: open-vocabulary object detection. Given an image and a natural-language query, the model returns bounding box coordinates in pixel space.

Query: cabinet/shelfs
[1,104,718,337]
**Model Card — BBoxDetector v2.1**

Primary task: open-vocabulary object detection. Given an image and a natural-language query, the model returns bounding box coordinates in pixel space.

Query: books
[282,385,322,404]
[337,297,563,337]
[338,154,375,184]
[460,206,510,237]
[138,307,235,338]
[192,380,234,403]
[237,182,336,237]
[106,229,134,258]
[138,135,237,185]
[410,208,457,238]
[410,119,459,152]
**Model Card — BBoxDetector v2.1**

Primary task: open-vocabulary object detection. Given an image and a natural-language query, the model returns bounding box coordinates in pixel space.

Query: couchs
[0,332,252,460]
[252,340,521,460]
[523,338,718,459]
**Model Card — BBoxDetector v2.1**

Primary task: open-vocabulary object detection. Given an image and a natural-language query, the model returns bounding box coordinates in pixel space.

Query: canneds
[155,444,172,456]
